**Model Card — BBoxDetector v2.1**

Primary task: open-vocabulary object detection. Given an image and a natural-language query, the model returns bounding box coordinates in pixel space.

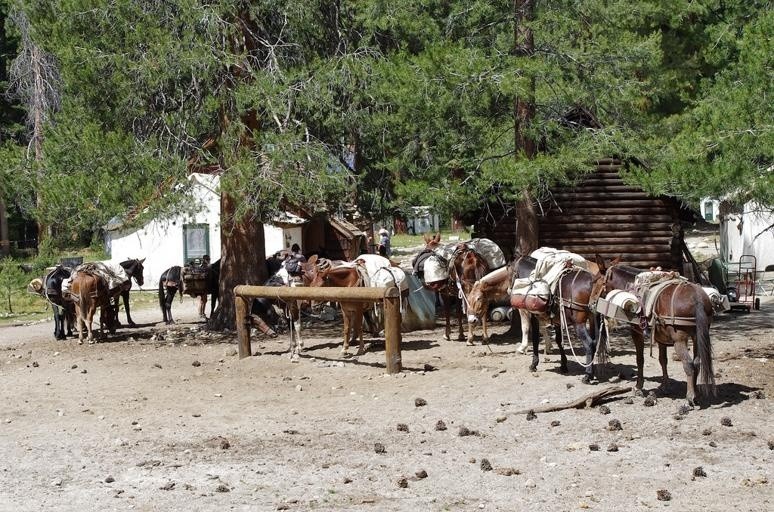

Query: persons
[291,243,306,263]
[379,228,391,259]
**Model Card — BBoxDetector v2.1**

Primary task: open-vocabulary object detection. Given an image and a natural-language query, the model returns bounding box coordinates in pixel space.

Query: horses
[43,257,146,345]
[158,258,221,325]
[588,253,718,411]
[413,230,609,383]
[248,251,409,360]
